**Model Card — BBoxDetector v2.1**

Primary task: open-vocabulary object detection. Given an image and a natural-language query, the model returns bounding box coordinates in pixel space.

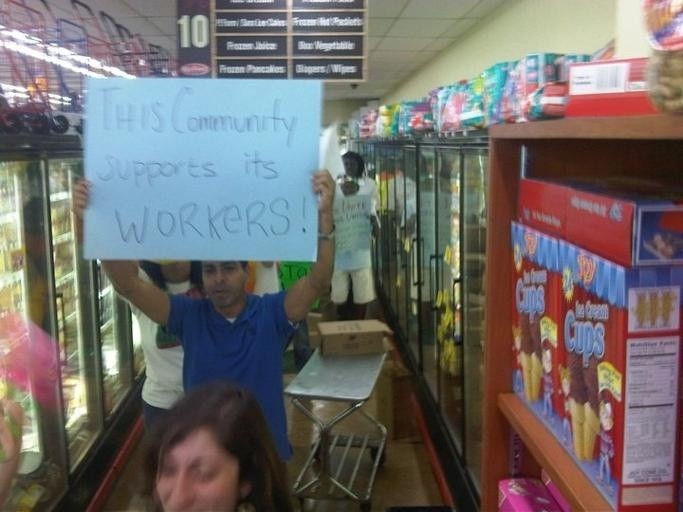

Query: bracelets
[316,227,336,240]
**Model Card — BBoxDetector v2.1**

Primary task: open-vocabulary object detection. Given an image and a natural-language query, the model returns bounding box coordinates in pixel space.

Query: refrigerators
[376,139,490,511]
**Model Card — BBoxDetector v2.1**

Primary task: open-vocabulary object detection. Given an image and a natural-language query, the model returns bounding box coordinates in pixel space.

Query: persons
[246,262,279,297]
[112,260,209,433]
[0,398,25,510]
[293,318,310,372]
[331,173,381,320]
[341,150,380,216]
[146,381,294,512]
[71,169,336,460]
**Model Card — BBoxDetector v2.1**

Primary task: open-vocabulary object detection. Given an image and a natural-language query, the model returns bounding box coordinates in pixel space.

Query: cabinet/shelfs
[482,118,683,512]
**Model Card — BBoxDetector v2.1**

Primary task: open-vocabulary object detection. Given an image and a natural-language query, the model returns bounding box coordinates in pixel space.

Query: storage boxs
[509,178,681,512]
[497,478,560,512]
[308,312,395,356]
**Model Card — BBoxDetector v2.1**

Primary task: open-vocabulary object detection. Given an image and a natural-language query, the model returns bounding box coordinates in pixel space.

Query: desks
[283,345,389,512]
